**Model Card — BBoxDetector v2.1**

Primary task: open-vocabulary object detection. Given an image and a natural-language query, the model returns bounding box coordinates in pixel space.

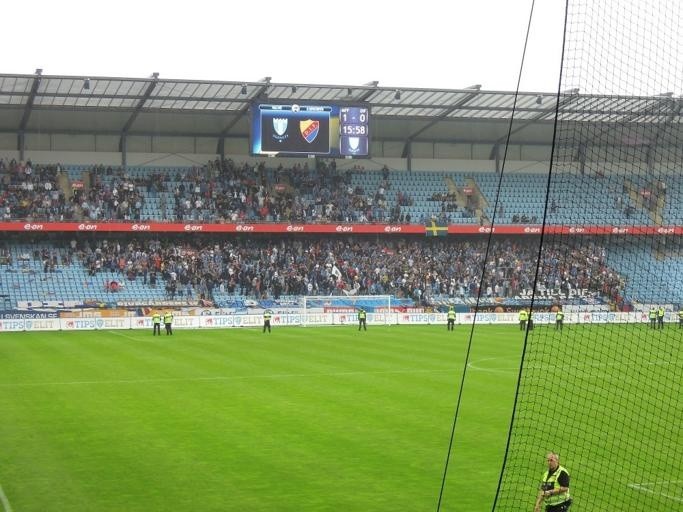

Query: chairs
[0,165,681,314]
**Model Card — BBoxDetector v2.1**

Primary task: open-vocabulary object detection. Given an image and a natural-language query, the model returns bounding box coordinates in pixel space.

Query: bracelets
[550,491,553,497]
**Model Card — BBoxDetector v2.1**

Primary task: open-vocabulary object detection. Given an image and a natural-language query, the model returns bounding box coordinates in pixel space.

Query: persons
[598,170,668,218]
[649,308,657,330]
[518,307,528,331]
[262,311,271,334]
[533,453,573,512]
[3,157,480,225]
[678,308,683,329]
[0,232,636,316]
[658,307,665,330]
[526,307,534,330]
[151,312,161,336]
[447,308,456,331]
[357,307,367,331]
[163,311,173,336]
[556,309,565,331]
[499,199,558,224]
